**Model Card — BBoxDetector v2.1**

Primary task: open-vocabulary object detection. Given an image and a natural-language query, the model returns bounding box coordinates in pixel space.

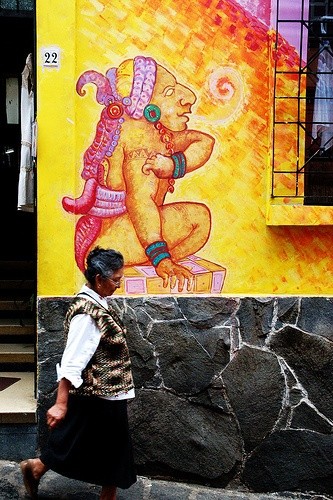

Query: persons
[21,246,136,500]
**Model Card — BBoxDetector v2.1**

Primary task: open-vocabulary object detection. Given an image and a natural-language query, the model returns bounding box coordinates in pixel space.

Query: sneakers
[21,460,38,498]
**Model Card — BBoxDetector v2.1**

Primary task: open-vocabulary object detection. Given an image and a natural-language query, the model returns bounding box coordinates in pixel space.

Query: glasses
[102,275,122,285]
